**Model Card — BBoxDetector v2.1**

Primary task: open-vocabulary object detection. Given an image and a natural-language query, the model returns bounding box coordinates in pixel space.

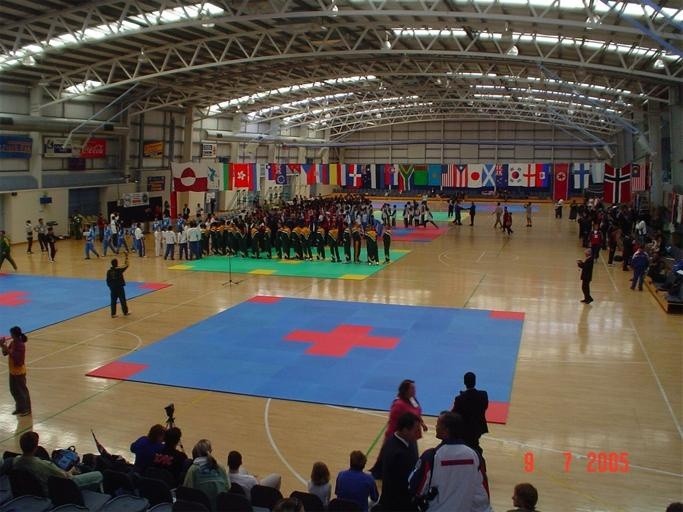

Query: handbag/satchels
[51,444,82,476]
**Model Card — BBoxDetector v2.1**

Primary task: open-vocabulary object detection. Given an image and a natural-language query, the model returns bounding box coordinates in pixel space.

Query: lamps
[508,45,518,56]
[138,46,146,63]
[22,51,36,65]
[381,31,391,50]
[201,9,214,27]
[328,0,338,17]
[652,50,663,69]
[585,12,602,29]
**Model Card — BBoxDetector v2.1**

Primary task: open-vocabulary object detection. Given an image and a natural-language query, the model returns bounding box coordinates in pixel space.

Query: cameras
[164,402,174,420]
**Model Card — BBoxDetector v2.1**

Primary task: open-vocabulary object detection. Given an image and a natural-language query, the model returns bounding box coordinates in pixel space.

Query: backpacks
[190,462,230,499]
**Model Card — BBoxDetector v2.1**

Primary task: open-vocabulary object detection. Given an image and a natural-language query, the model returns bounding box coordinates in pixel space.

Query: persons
[569,197,683,304]
[555,198,563,217]
[0,230,17,272]
[154,192,392,264]
[0,326,31,417]
[391,201,439,229]
[106,251,132,318]
[81,209,148,259]
[491,202,514,236]
[665,501,683,512]
[523,202,532,227]
[24,220,35,255]
[10,431,103,494]
[369,372,495,512]
[46,227,64,263]
[33,218,48,251]
[130,424,379,511]
[422,192,476,226]
[507,482,540,512]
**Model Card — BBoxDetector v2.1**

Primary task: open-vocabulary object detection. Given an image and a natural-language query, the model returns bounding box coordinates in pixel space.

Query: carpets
[0,272,172,342]
[86,299,520,425]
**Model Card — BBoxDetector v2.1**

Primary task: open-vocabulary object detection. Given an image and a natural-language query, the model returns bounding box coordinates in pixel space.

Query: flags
[630,163,646,191]
[248,162,261,193]
[300,163,317,186]
[170,161,209,192]
[207,163,219,189]
[317,160,606,201]
[604,162,633,205]
[219,162,233,191]
[123,191,149,207]
[268,163,277,181]
[233,163,248,188]
[276,164,287,184]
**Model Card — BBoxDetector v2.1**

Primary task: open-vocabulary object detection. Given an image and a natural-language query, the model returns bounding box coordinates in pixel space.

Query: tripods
[221,254,239,289]
[165,420,185,455]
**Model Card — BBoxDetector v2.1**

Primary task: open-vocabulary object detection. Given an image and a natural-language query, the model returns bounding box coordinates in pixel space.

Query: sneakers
[19,410,32,417]
[11,409,22,416]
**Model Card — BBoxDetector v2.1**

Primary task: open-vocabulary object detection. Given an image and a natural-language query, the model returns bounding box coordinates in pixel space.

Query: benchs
[660,247,682,272]
